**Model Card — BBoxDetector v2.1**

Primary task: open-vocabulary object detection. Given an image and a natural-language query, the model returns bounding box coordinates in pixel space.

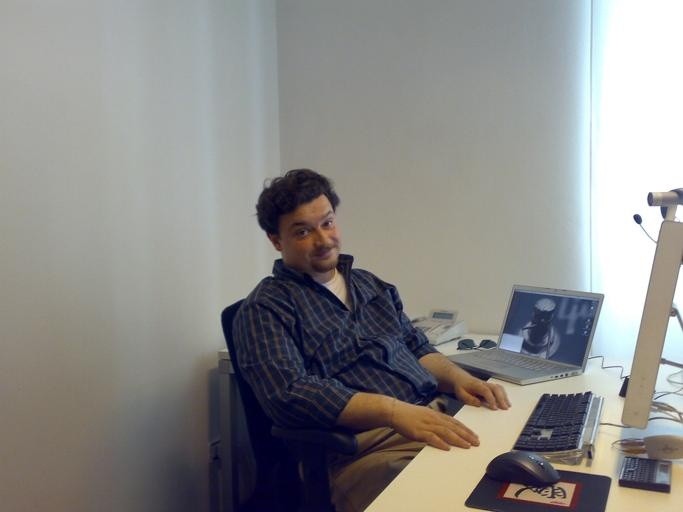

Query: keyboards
[511,391,604,459]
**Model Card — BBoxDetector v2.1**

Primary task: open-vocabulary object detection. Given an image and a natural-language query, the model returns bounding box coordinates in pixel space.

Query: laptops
[445,285,604,386]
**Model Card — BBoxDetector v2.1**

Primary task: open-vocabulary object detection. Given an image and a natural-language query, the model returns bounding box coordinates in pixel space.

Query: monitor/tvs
[621,221,683,460]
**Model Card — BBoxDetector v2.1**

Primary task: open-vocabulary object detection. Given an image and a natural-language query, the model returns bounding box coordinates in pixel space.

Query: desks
[216,334,683,512]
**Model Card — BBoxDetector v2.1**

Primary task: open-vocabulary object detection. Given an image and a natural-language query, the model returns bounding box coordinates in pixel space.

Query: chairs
[220,299,358,511]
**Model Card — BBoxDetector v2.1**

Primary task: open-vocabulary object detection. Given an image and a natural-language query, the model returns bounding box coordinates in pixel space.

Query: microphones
[634,214,658,243]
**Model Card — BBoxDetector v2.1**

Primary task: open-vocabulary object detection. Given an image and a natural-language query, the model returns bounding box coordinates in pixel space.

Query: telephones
[411,310,467,345]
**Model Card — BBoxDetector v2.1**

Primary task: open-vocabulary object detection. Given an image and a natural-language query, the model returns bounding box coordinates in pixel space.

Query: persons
[231,169,511,512]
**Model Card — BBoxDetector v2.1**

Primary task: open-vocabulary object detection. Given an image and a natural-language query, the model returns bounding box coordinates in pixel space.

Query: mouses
[486,451,560,487]
